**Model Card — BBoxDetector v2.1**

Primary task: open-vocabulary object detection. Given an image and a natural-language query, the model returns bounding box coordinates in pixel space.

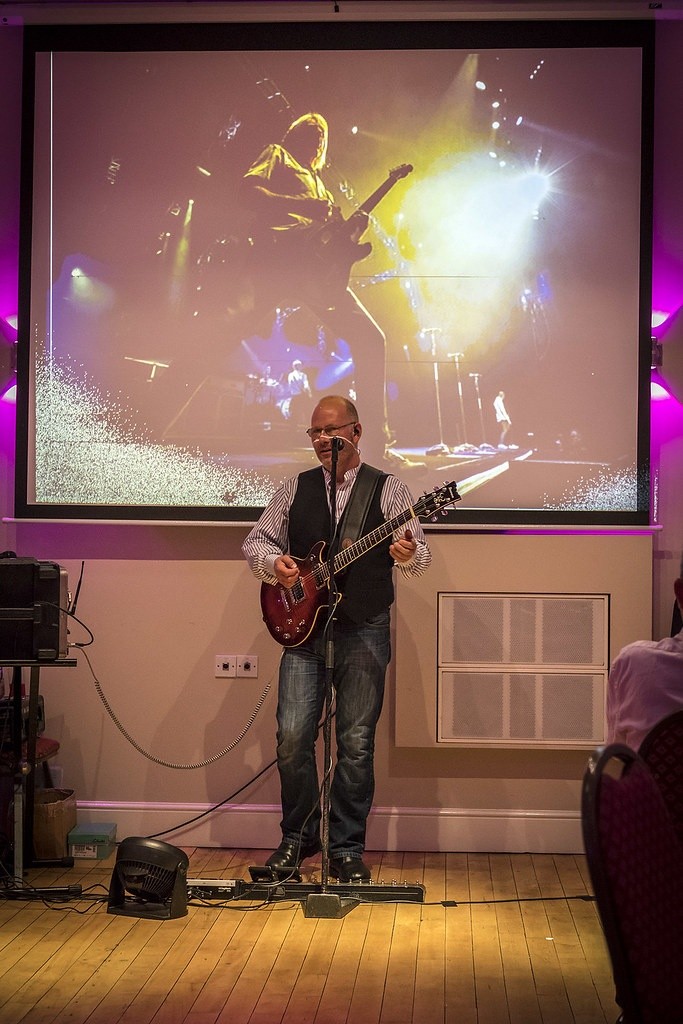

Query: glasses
[306,421,356,440]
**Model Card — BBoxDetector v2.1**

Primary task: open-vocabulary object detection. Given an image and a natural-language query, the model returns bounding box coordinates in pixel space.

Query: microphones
[331,437,344,450]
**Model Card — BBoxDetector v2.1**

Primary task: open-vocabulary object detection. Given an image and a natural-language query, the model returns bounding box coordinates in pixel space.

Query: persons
[245,396,432,881]
[240,114,404,470]
[608,569,683,755]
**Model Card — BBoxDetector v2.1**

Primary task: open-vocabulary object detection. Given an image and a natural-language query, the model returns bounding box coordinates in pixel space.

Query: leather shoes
[265,841,322,867]
[329,856,370,883]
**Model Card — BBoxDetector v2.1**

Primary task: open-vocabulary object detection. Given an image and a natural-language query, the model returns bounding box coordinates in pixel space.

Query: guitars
[279,165,414,312]
[257,479,461,653]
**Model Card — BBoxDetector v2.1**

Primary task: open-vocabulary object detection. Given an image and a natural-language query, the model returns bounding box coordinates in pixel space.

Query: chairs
[581,709,683,1024]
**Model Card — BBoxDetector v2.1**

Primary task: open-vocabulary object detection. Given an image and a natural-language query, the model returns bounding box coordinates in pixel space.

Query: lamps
[106,836,189,922]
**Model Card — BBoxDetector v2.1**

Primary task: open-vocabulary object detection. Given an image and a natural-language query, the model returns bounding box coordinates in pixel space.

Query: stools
[5,737,60,861]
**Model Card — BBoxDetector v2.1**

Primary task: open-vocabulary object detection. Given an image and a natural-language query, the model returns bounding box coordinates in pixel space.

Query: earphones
[354,429,359,436]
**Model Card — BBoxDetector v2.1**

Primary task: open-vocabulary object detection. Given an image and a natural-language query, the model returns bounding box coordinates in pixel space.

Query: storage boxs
[66,822,117,859]
[8,787,77,860]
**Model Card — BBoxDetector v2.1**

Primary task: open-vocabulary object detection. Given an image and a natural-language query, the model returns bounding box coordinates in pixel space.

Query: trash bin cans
[34,787,77,861]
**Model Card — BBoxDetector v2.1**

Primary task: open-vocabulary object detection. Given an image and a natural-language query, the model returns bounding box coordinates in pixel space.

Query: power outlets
[214,654,237,677]
[236,655,257,677]
